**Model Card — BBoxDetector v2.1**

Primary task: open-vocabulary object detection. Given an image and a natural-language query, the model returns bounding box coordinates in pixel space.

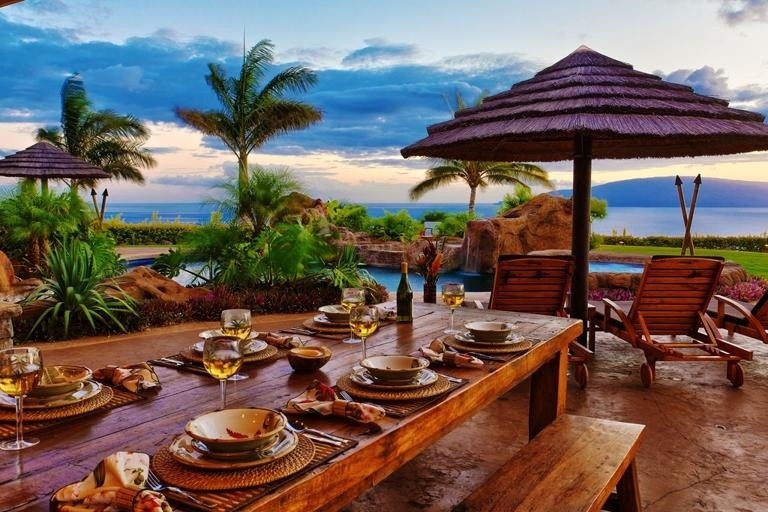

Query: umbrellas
[0,141,112,196]
[399,46,768,351]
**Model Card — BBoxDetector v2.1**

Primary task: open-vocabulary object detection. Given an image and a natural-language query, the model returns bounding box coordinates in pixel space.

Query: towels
[274,379,385,425]
[93,361,163,398]
[376,305,396,321]
[48,451,173,512]
[257,330,299,349]
[408,337,484,368]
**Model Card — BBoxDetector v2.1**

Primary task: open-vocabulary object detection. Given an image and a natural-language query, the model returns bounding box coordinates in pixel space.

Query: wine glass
[203,334,242,407]
[1,346,41,452]
[442,281,467,337]
[343,289,378,363]
[220,307,252,380]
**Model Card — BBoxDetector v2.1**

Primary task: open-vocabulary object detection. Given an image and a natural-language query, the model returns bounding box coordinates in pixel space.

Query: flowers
[414,237,450,285]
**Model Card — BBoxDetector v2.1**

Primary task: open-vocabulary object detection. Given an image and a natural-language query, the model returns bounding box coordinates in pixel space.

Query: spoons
[287,418,351,444]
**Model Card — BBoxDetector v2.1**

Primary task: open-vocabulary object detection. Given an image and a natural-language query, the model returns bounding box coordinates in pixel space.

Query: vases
[424,284,436,304]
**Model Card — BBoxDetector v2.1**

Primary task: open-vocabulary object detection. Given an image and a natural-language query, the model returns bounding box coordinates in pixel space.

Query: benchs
[450,414,648,512]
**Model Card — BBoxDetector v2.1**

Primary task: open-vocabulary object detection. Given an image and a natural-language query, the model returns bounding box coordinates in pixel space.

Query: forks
[142,467,216,508]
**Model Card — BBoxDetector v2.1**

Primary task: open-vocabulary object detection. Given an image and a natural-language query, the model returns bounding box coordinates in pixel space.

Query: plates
[195,329,268,355]
[2,361,100,411]
[313,303,368,327]
[347,354,439,390]
[455,321,527,347]
[171,410,301,469]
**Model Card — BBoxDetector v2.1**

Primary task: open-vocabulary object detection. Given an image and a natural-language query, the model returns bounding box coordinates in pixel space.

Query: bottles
[394,258,415,324]
